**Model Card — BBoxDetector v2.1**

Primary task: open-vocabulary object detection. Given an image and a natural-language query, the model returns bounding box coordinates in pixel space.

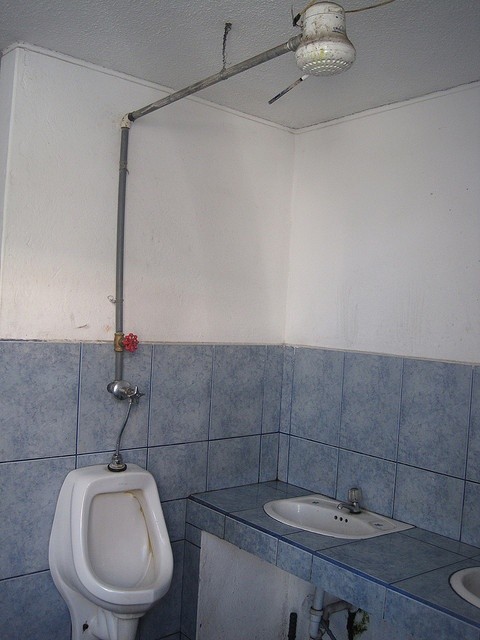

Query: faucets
[337,488,364,514]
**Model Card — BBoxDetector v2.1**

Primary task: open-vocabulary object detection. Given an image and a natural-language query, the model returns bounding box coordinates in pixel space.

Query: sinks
[449,565,480,609]
[262,493,417,539]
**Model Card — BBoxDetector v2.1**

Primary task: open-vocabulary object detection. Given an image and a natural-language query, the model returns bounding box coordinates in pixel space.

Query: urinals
[53,463,174,639]
